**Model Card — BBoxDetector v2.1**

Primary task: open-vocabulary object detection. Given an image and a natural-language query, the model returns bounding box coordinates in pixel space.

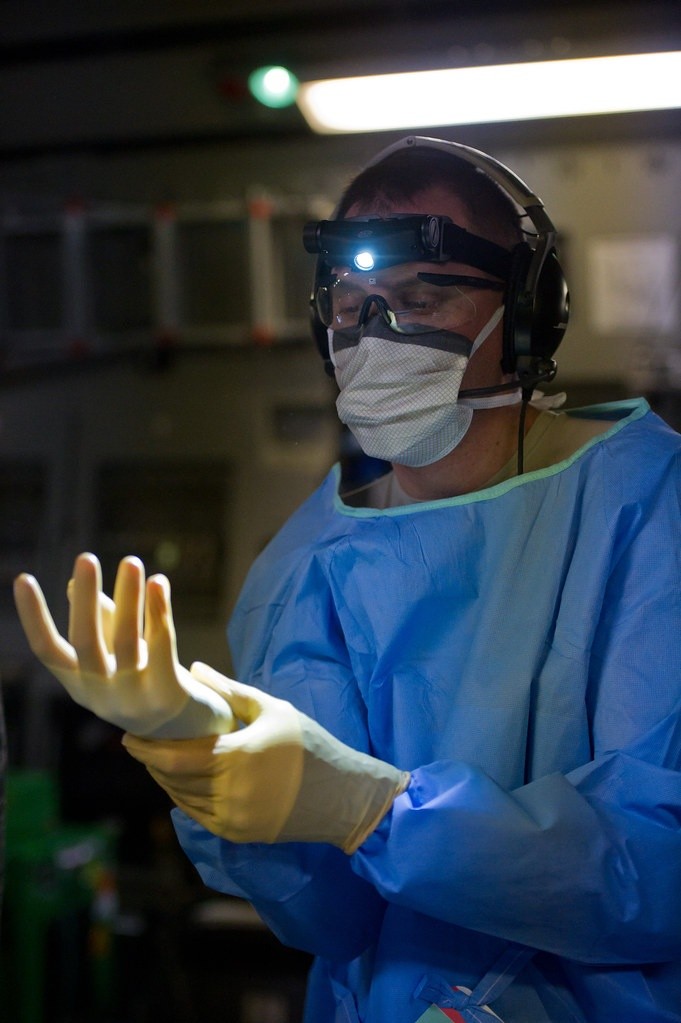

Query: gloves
[14,551,237,742]
[122,661,412,855]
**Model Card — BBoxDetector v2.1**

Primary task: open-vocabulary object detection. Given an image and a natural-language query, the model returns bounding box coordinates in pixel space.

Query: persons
[14,136,681,1023]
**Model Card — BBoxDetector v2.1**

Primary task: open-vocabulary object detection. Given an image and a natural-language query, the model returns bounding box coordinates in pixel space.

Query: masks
[327,306,523,469]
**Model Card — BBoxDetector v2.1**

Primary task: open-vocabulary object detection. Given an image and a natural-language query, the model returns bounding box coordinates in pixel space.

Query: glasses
[312,272,505,336]
